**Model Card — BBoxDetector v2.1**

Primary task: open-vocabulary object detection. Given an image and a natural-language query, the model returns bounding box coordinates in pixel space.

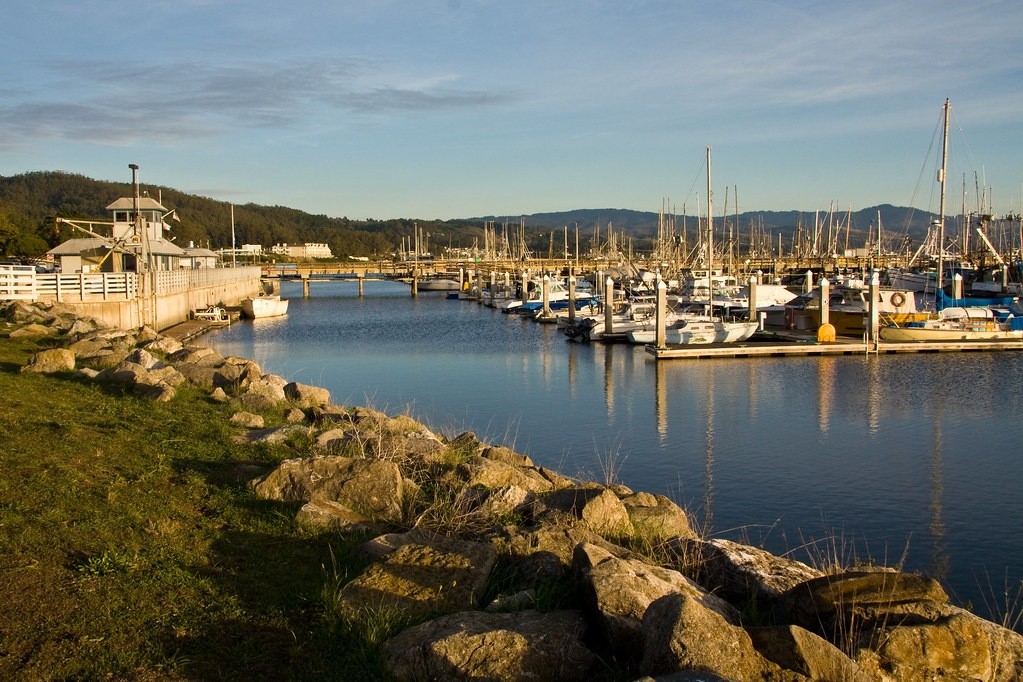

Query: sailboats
[394,98,1023,344]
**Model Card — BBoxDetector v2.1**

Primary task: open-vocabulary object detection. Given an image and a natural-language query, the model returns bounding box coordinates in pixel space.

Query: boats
[240,297,290,319]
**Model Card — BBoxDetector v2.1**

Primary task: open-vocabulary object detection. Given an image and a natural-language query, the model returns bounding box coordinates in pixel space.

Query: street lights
[129,163,139,273]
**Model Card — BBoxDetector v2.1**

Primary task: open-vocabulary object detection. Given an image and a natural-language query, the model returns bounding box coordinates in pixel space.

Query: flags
[163,221,172,231]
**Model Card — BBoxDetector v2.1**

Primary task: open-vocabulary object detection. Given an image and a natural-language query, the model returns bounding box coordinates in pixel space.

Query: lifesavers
[890,292,906,307]
[817,270,827,278]
